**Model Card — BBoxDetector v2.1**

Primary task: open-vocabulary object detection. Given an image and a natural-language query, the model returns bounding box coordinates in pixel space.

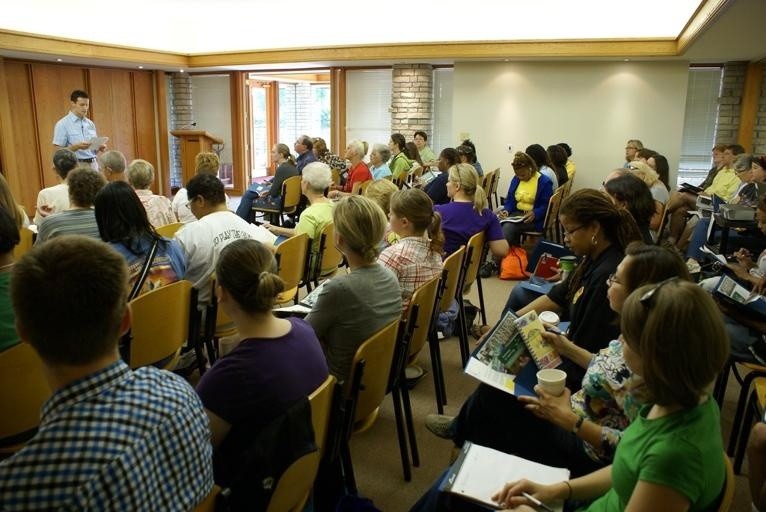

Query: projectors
[718,203,755,221]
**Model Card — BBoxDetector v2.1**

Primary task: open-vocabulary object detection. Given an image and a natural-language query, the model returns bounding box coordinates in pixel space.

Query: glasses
[734,168,745,176]
[625,147,638,150]
[626,163,638,171]
[609,273,626,288]
[184,196,195,209]
[639,274,679,311]
[564,225,583,238]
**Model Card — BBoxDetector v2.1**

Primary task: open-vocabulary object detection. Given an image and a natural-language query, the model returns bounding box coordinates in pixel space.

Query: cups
[536,310,560,328]
[536,368,567,398]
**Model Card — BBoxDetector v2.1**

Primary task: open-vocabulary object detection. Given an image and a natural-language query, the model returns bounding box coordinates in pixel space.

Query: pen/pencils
[727,254,754,259]
[546,329,566,336]
[522,491,554,512]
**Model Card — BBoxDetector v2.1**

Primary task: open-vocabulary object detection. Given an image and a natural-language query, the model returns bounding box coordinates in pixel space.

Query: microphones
[179,122,196,129]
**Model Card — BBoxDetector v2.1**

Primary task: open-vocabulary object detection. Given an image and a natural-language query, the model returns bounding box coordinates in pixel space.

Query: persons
[407,278,730,512]
[424,241,691,478]
[53,90,106,169]
[1,233,214,512]
[196,241,328,512]
[2,132,766,391]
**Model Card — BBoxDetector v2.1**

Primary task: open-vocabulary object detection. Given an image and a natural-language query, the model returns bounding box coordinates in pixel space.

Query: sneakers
[491,255,501,274]
[478,262,494,278]
[424,414,456,440]
[663,239,701,274]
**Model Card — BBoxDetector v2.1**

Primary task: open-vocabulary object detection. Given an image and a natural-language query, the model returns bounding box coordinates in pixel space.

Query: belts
[79,158,96,163]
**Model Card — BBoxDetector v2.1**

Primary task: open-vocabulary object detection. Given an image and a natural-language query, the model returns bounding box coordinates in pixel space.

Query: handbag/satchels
[701,262,753,291]
[304,436,382,512]
[499,245,530,280]
[452,299,481,336]
[534,253,561,280]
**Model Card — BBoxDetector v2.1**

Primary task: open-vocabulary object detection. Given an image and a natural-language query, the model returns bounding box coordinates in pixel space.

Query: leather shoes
[281,220,296,228]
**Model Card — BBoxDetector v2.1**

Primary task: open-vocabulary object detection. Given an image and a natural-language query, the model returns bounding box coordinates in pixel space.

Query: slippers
[469,324,489,340]
